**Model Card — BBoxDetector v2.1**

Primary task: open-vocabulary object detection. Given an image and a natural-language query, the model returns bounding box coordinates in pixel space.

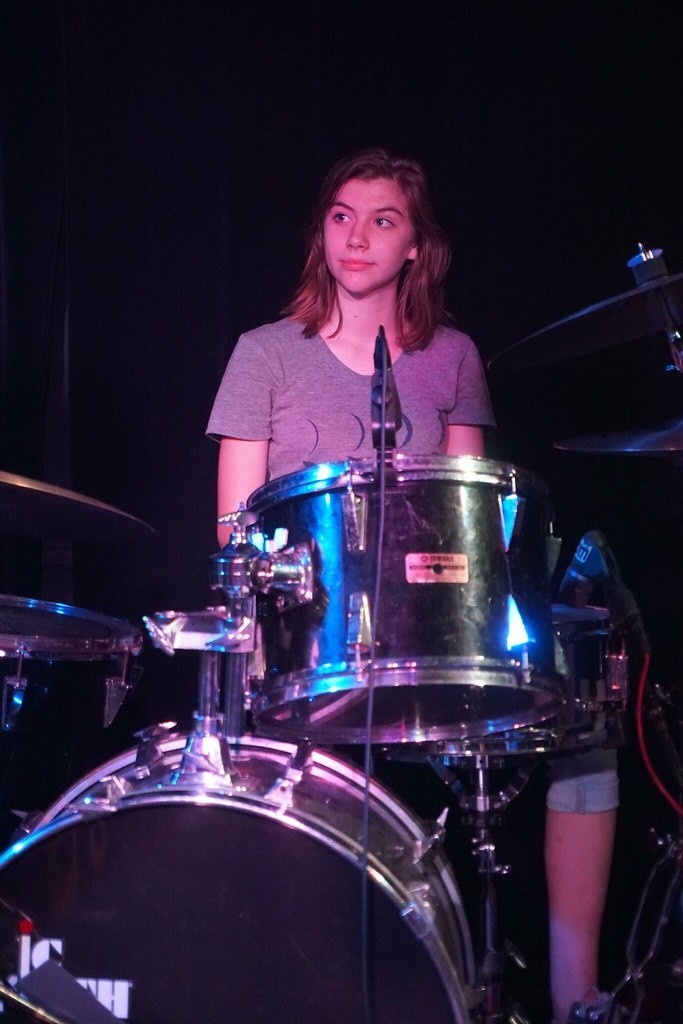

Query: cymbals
[0,470,159,550]
[483,272,683,376]
[552,414,683,454]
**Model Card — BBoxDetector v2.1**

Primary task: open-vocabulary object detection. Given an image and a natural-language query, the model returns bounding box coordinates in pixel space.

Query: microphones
[604,583,650,659]
[371,333,401,453]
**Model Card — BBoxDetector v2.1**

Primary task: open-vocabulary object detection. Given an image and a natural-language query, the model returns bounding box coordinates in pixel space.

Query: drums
[381,597,630,771]
[0,730,481,1024]
[1,591,147,814]
[238,450,571,751]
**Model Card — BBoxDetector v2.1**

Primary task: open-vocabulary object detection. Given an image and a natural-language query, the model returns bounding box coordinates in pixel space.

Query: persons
[205,149,619,1024]
